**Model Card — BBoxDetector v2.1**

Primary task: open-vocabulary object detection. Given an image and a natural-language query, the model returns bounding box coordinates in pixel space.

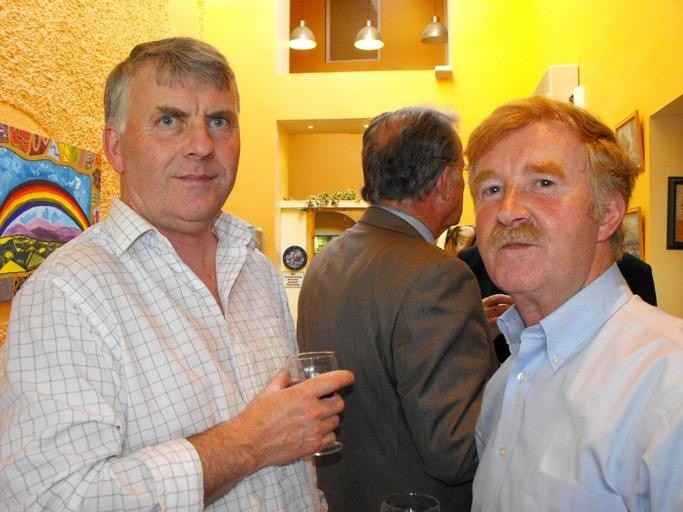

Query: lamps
[420,0,448,44]
[289,0,317,50]
[353,0,385,51]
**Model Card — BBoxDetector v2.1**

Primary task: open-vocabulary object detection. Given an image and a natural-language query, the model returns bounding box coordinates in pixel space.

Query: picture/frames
[622,206,645,263]
[666,176,683,249]
[614,110,645,172]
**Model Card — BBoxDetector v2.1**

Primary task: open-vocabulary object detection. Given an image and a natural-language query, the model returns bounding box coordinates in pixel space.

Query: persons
[444,224,657,363]
[296,107,514,512]
[463,96,683,512]
[0,37,354,512]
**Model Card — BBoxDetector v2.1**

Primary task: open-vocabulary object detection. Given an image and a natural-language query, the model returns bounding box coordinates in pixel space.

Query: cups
[375,491,442,511]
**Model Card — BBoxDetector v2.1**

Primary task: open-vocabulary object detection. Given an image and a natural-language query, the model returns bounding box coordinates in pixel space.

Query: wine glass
[281,350,345,459]
